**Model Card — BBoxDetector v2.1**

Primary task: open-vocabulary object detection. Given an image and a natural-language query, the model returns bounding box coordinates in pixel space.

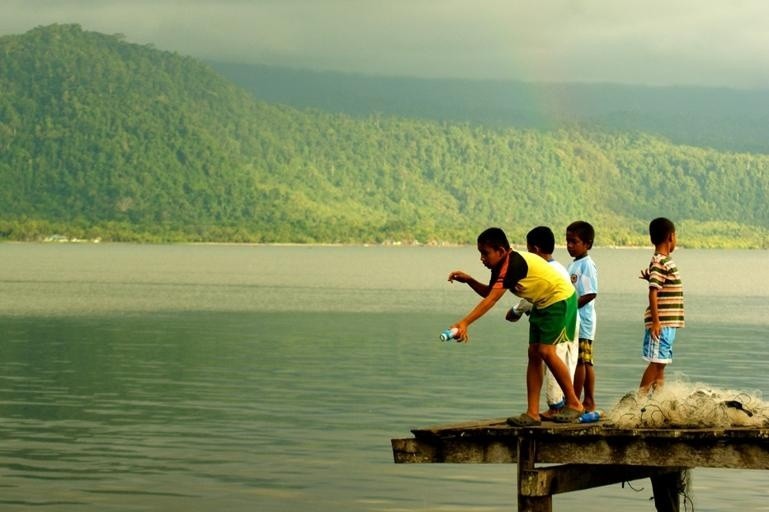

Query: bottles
[439,327,459,342]
[575,408,606,423]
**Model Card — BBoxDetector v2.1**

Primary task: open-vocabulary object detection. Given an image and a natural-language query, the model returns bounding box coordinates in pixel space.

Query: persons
[635,216,686,396]
[503,225,581,421]
[444,227,586,427]
[565,219,599,414]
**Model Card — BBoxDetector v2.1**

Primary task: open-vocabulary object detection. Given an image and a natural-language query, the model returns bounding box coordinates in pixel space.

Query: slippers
[507,407,586,426]
